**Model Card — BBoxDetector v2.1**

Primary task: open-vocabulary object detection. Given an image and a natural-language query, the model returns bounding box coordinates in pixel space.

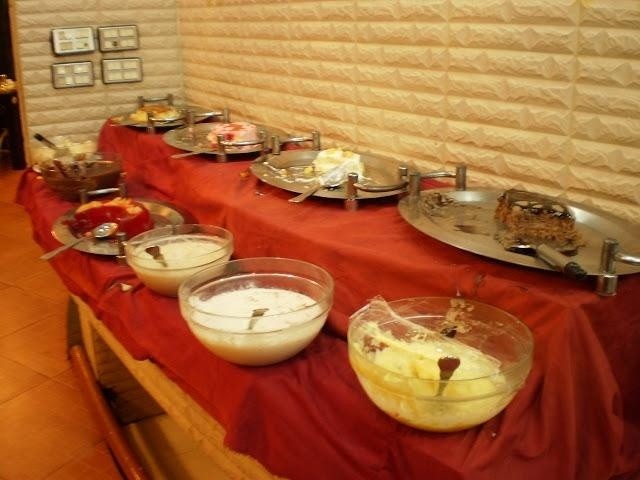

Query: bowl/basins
[125,223,235,298]
[346,294,535,435]
[38,150,124,198]
[177,256,335,368]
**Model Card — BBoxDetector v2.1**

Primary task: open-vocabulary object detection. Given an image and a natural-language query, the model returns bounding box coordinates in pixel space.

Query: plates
[51,196,185,257]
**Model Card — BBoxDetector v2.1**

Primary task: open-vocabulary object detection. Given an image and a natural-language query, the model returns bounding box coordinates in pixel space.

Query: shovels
[33,132,70,159]
[497,228,588,280]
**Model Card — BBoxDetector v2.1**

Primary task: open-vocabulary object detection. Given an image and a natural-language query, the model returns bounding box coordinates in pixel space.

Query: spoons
[39,221,118,261]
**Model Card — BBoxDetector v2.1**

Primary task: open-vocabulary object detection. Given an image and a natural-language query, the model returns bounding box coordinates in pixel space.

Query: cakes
[495,189,578,242]
[206,122,261,151]
[74,196,155,242]
[129,104,179,123]
[312,148,365,174]
